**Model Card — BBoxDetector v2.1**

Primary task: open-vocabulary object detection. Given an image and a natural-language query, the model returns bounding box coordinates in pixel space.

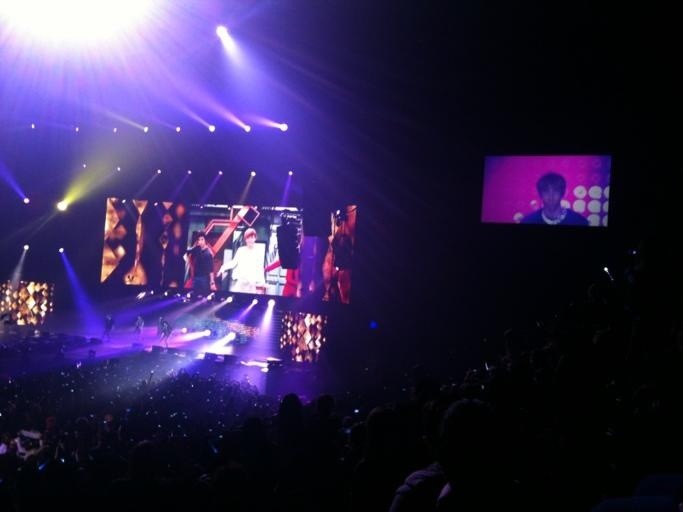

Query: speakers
[277,226,300,269]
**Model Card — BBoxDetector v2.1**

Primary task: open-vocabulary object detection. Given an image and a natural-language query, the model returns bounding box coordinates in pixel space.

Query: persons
[160,321,175,347]
[102,312,117,343]
[133,312,145,342]
[1,356,509,511]
[184,228,266,294]
[520,173,589,226]
